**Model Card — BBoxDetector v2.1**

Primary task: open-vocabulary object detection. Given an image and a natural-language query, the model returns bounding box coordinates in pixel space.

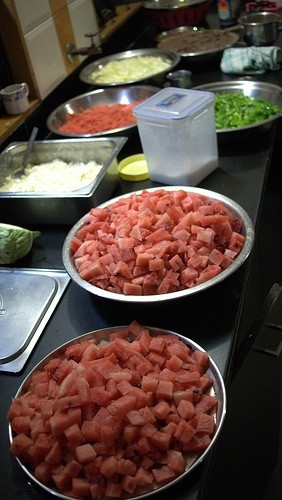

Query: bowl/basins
[79,48,181,89]
[245,1,279,13]
[46,84,163,144]
[62,186,255,306]
[156,29,240,66]
[189,80,282,144]
[237,11,280,46]
[142,0,213,30]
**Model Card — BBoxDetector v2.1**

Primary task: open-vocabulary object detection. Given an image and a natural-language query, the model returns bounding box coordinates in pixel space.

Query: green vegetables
[213,92,281,129]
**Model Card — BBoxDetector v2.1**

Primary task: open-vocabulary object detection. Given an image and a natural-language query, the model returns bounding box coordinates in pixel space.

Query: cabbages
[91,55,171,84]
[0,223,33,264]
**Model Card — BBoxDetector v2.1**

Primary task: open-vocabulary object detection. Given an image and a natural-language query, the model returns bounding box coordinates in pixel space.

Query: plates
[155,25,205,42]
[7,326,226,500]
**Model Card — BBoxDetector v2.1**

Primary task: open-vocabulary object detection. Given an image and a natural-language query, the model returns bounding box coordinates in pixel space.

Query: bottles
[216,0,238,26]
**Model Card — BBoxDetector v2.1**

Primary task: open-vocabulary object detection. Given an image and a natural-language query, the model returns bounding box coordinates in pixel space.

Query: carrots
[58,101,143,133]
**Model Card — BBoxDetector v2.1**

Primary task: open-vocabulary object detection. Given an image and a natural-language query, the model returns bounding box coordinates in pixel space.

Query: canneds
[217,0,234,24]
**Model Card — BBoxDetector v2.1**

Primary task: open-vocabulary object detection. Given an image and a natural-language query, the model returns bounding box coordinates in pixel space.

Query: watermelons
[69,188,246,296]
[6,323,218,499]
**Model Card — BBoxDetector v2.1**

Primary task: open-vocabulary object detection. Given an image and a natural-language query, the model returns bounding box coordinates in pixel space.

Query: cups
[0,83,30,115]
[166,70,192,88]
